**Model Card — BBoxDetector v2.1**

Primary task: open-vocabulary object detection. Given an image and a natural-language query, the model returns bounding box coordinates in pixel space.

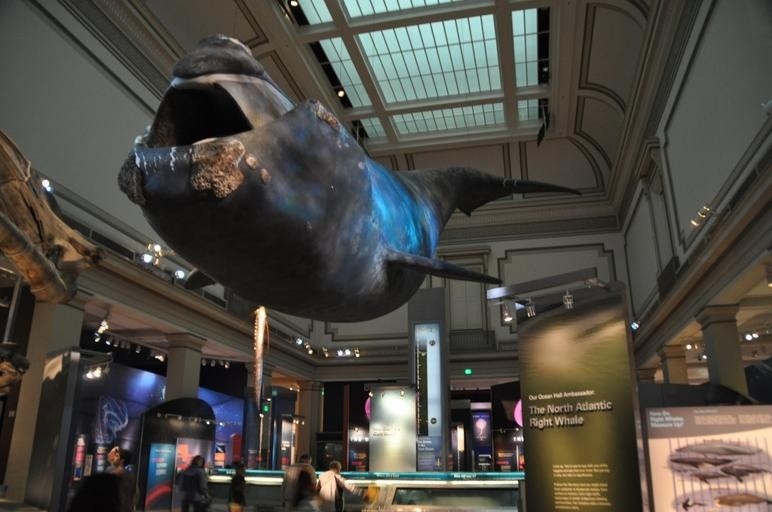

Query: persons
[315,460,358,512]
[229,463,248,512]
[176,455,211,511]
[104,446,120,473]
[112,450,132,474]
[282,452,318,512]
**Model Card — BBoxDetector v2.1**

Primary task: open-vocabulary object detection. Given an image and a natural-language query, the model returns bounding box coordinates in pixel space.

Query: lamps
[201,358,232,369]
[500,276,607,323]
[690,202,724,230]
[138,240,186,280]
[294,337,362,360]
[91,304,165,365]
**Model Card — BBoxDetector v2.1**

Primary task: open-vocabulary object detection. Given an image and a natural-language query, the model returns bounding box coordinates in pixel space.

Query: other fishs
[714,491,772,506]
[671,443,772,483]
